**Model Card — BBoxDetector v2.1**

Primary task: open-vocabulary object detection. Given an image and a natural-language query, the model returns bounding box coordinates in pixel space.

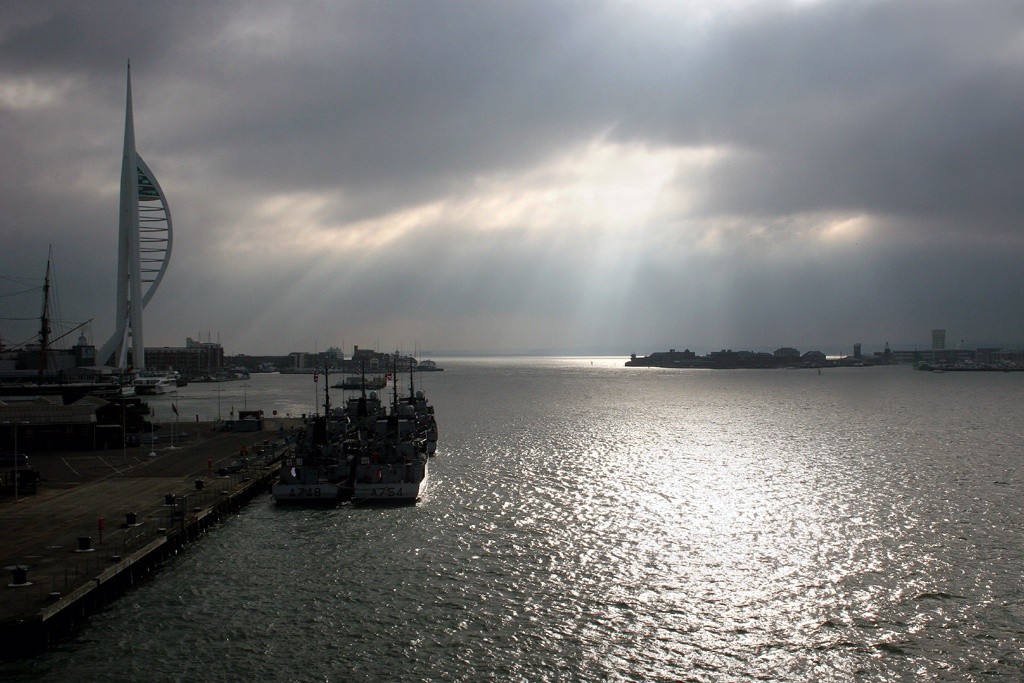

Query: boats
[272,352,437,506]
[132,372,178,396]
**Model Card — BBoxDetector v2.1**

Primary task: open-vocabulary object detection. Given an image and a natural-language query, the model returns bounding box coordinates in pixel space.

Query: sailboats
[0,242,150,425]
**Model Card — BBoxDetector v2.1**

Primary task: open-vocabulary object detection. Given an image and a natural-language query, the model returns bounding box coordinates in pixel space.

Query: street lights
[114,403,135,464]
[2,421,30,502]
[211,389,225,420]
[168,396,185,442]
[240,385,251,410]
